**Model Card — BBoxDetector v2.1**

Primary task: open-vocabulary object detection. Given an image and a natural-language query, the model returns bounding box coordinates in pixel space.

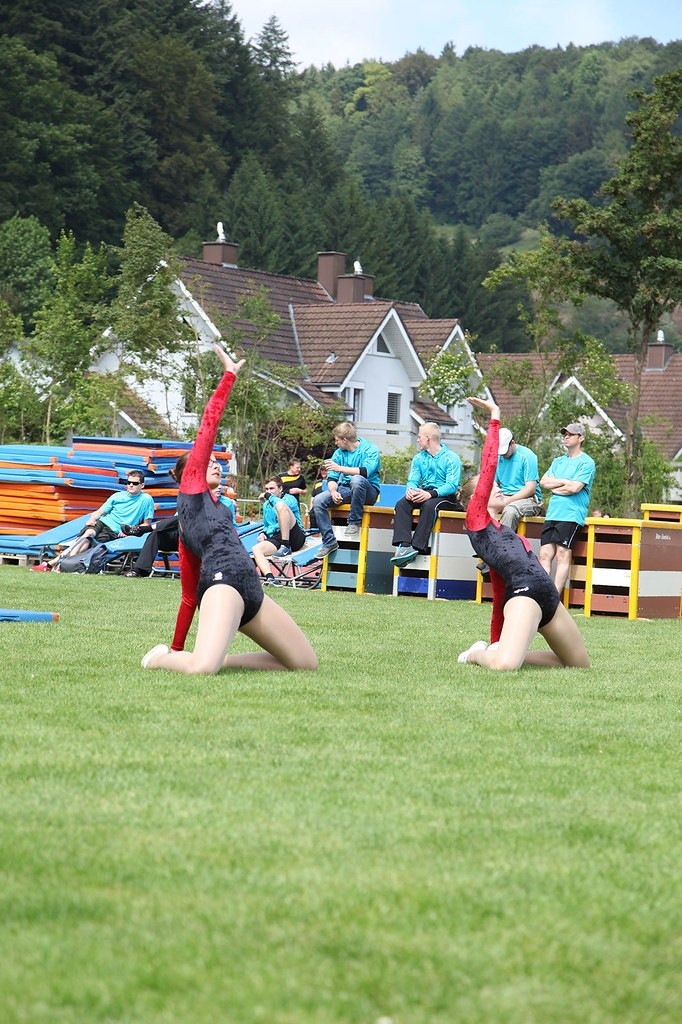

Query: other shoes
[477,561,490,576]
[488,642,499,650]
[458,640,489,665]
[140,644,169,668]
[124,570,143,577]
[121,525,143,537]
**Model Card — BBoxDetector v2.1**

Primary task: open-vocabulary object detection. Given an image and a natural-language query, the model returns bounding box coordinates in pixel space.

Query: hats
[560,424,586,439]
[497,428,512,455]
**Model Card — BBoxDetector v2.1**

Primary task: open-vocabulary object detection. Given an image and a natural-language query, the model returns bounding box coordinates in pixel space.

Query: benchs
[47,512,323,590]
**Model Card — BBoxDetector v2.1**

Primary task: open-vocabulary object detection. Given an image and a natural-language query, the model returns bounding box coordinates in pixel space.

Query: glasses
[126,480,141,486]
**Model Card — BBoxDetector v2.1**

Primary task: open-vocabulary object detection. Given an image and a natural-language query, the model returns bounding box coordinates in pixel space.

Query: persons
[389,422,462,567]
[309,461,327,534]
[278,457,308,516]
[120,485,237,578]
[30,469,155,573]
[312,422,382,559]
[140,343,321,674]
[476,426,544,576]
[539,423,596,595]
[456,396,591,671]
[251,475,306,587]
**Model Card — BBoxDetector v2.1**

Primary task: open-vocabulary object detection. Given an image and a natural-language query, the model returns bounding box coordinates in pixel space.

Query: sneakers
[272,545,292,558]
[390,546,418,567]
[55,564,64,573]
[263,578,283,588]
[315,542,339,558]
[345,523,359,535]
[29,561,52,572]
